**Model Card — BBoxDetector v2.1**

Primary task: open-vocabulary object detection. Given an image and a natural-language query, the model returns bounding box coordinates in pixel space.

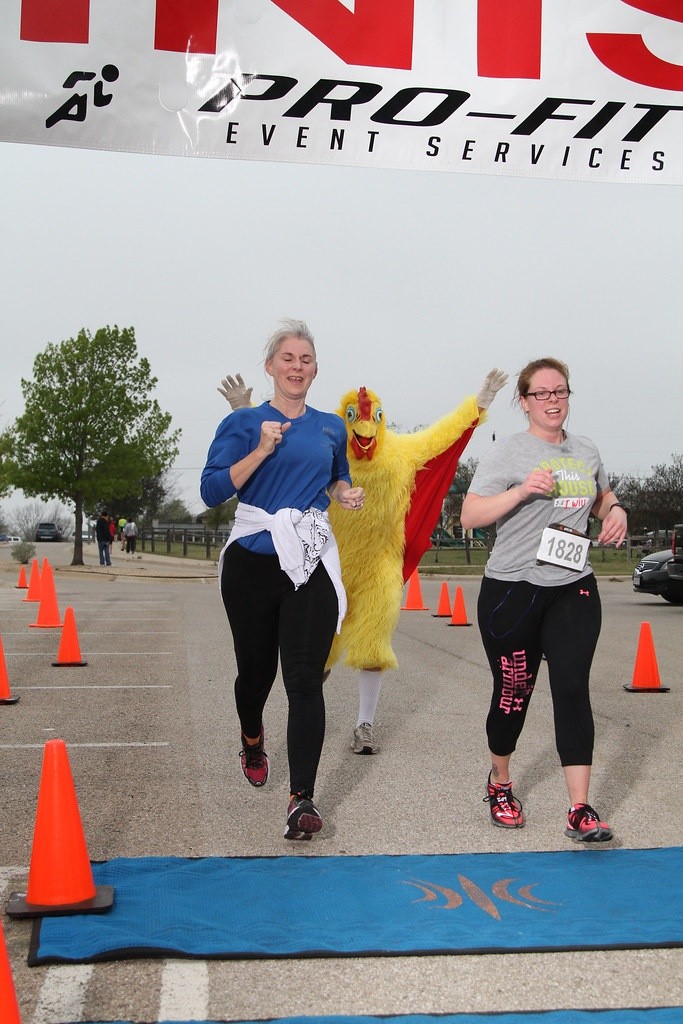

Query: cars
[0,533,23,544]
[631,548,683,607]
[68,530,93,543]
[430,528,466,548]
[588,529,672,550]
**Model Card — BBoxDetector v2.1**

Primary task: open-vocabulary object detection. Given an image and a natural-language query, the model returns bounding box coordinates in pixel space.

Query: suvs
[35,523,63,542]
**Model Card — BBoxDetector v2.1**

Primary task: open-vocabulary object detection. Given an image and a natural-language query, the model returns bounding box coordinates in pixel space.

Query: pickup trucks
[666,524,683,581]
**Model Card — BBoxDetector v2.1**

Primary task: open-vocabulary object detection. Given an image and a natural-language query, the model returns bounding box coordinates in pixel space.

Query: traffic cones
[398,567,429,611]
[28,558,64,628]
[14,564,29,589]
[446,586,474,627]
[622,621,671,693]
[4,738,115,920]
[21,559,42,602]
[431,581,453,618]
[1,918,25,1024]
[0,634,20,705]
[51,607,87,667]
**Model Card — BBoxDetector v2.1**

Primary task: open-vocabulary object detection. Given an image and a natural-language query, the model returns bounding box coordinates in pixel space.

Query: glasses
[524,389,571,400]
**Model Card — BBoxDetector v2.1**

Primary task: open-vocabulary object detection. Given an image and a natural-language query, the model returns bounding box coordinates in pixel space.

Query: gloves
[217,374,253,410]
[478,369,509,409]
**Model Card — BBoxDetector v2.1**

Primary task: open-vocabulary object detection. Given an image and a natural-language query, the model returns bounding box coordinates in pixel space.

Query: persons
[217,366,509,753]
[200,319,365,841]
[459,358,628,842]
[96,511,137,567]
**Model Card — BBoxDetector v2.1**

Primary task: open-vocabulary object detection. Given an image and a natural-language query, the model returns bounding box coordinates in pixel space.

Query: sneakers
[563,802,613,843]
[351,722,381,755]
[239,722,268,787]
[283,789,323,840]
[482,769,526,829]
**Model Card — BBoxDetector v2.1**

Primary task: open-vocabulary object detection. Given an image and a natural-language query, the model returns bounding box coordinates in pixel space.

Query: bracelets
[610,503,630,517]
